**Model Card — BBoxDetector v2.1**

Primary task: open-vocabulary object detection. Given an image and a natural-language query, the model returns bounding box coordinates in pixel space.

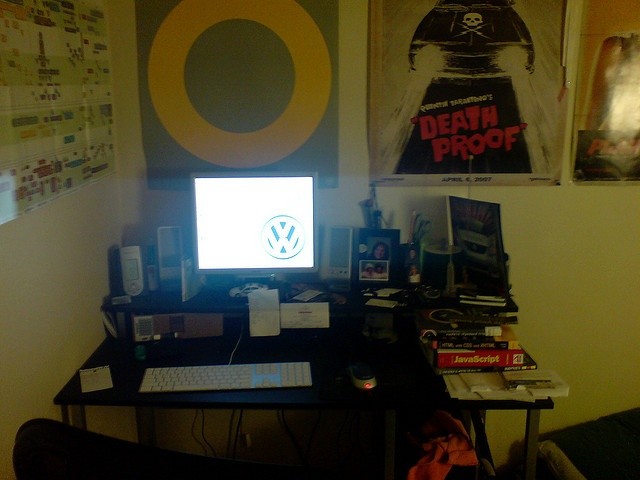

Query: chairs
[535,406,639,480]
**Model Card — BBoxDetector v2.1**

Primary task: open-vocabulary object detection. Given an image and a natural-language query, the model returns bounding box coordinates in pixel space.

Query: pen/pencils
[411,211,431,243]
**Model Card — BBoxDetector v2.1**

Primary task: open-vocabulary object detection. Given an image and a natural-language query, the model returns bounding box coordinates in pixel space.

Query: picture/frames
[368,3,563,187]
[353,228,402,290]
[136,2,337,191]
[568,0,640,184]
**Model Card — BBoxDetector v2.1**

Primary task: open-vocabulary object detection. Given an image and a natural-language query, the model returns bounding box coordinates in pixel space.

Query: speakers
[319,226,354,280]
[156,225,188,281]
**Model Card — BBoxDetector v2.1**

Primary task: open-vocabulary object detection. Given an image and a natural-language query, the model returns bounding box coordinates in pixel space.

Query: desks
[54,337,556,479]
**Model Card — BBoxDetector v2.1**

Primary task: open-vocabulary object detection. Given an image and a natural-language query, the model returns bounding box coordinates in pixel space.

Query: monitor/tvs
[191,171,321,276]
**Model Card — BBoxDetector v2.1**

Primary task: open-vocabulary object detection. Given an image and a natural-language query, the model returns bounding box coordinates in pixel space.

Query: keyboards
[138,361,312,394]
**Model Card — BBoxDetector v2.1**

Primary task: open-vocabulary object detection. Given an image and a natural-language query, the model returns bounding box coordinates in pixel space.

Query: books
[425,325,538,374]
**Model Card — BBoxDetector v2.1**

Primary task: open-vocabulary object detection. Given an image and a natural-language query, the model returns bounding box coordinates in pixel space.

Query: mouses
[349,366,380,392]
